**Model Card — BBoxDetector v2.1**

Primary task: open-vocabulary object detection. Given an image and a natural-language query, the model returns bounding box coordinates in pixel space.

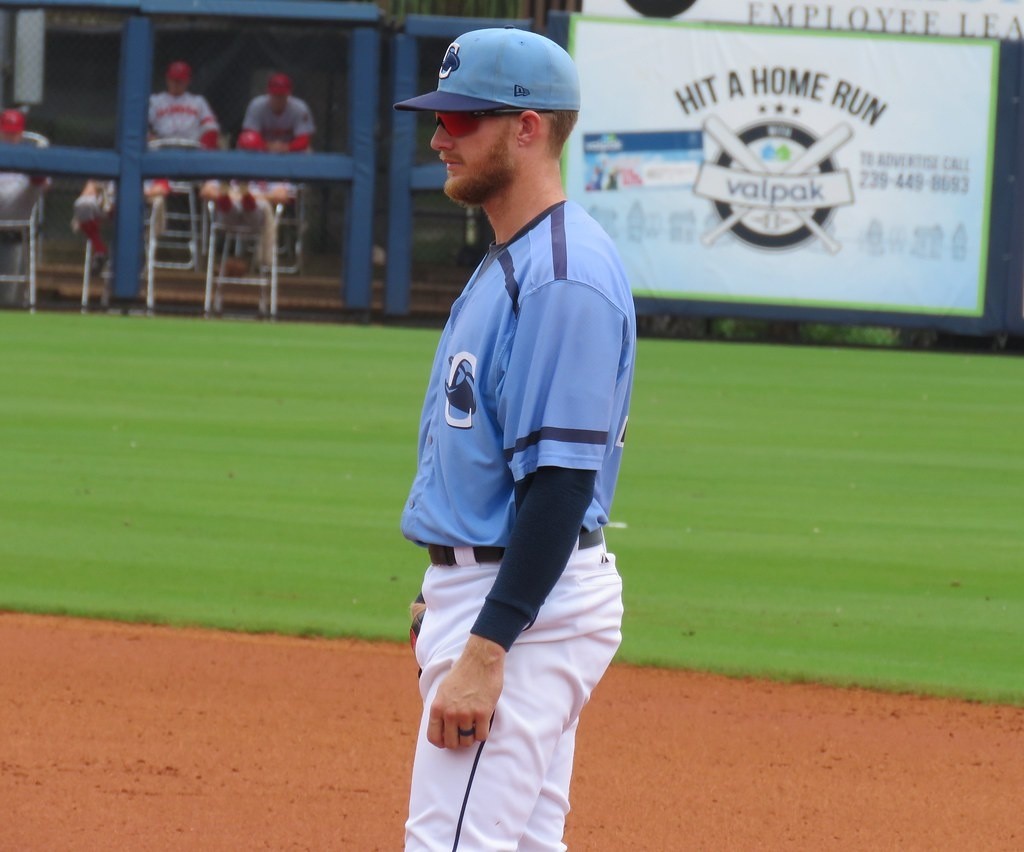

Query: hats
[392,24,580,112]
[238,131,267,150]
[166,63,192,82]
[0,109,26,134]
[267,75,293,96]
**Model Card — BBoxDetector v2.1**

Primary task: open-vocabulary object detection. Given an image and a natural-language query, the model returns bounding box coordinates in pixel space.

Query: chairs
[0,129,311,320]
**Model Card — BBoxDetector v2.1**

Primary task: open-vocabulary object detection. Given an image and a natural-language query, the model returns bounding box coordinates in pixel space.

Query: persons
[0,109,51,305]
[391,27,636,852]
[71,61,317,277]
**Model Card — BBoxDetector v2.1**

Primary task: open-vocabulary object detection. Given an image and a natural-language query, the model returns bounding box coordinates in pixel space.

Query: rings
[459,726,475,736]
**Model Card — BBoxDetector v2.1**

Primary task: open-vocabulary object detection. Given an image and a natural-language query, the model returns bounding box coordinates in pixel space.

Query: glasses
[434,108,554,139]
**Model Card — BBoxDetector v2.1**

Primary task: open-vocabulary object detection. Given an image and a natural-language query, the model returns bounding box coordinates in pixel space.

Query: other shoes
[88,254,108,278]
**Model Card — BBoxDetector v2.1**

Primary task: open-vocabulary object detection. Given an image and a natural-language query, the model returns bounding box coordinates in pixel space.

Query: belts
[428,525,604,566]
